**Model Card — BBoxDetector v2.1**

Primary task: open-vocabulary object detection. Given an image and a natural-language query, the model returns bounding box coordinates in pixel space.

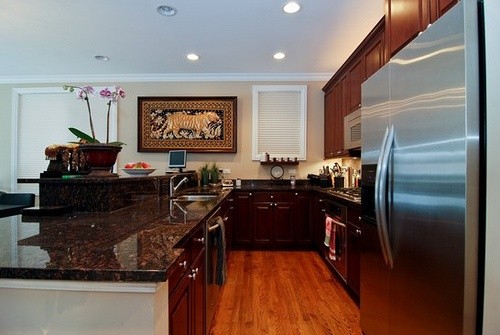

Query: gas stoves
[329,187,361,201]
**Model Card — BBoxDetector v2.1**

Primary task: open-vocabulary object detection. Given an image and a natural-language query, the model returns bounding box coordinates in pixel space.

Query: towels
[214,216,227,286]
[323,217,337,261]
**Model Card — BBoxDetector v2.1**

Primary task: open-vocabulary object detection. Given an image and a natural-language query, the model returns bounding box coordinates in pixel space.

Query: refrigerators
[359,0,499,335]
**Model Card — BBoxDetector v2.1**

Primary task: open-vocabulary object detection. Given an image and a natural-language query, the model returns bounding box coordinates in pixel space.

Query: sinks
[186,192,221,197]
[172,195,217,201]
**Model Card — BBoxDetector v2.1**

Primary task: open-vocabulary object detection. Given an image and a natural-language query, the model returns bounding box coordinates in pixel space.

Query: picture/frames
[137,96,237,154]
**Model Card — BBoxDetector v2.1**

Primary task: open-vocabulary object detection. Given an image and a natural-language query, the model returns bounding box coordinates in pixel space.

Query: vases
[78,145,123,177]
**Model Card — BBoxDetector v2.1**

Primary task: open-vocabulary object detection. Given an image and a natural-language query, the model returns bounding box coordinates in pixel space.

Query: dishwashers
[205,207,226,334]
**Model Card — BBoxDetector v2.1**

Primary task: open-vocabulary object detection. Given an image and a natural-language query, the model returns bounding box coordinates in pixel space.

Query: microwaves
[343,108,362,156]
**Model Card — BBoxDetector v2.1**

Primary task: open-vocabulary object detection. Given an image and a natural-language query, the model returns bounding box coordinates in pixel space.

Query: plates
[122,168,156,176]
[270,165,284,179]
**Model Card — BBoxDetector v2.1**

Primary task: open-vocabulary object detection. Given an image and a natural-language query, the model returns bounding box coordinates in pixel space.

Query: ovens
[324,195,349,287]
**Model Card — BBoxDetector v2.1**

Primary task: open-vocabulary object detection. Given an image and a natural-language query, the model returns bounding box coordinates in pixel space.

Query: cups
[320,178,329,188]
[334,177,344,189]
[265,154,298,162]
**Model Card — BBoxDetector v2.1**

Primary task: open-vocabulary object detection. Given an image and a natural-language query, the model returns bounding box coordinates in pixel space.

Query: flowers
[63,84,128,146]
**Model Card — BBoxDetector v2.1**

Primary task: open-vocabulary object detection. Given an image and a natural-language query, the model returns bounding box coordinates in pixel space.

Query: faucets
[170,174,189,198]
[169,199,188,218]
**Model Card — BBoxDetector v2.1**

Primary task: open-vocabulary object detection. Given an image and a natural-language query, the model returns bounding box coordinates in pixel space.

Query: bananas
[133,163,144,168]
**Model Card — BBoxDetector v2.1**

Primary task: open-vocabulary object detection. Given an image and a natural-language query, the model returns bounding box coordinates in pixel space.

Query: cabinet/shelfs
[231,191,324,254]
[386,0,460,63]
[324,30,386,159]
[168,190,231,335]
[347,207,362,310]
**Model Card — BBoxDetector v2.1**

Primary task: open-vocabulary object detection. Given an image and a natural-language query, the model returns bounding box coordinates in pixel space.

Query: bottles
[352,169,361,187]
[236,178,241,186]
